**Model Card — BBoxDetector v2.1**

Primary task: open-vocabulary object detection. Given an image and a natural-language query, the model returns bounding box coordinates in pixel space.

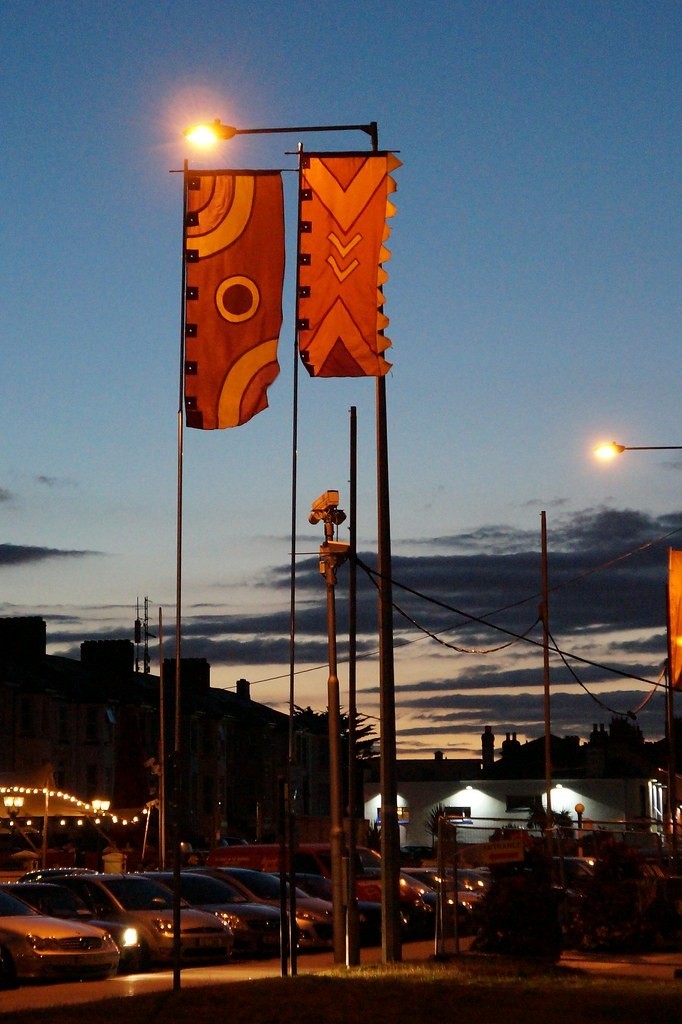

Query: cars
[203,843,492,947]
[553,854,596,877]
[16,867,233,973]
[1,883,121,991]
[131,867,334,959]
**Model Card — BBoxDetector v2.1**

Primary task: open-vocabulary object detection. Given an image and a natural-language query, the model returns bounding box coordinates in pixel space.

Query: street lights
[190,115,403,962]
